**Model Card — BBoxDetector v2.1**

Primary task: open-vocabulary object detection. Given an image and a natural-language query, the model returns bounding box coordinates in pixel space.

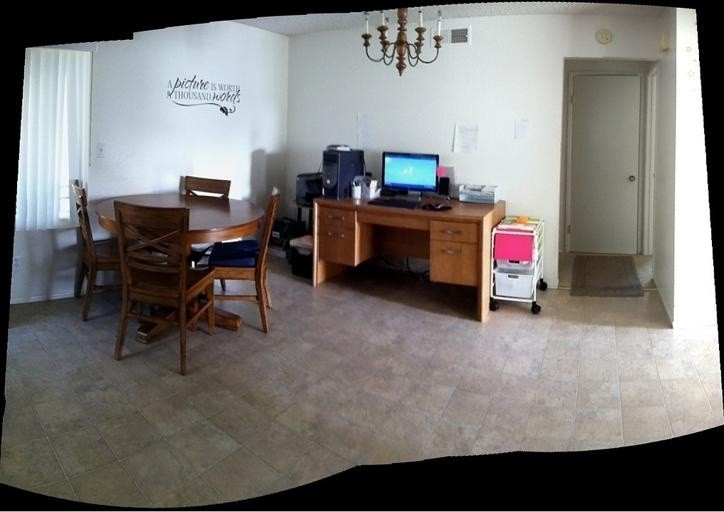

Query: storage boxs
[291,235,322,279]
[493,268,542,298]
[494,224,540,269]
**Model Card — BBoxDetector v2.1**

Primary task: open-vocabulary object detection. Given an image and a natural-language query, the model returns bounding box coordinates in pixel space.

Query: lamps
[362,9,442,76]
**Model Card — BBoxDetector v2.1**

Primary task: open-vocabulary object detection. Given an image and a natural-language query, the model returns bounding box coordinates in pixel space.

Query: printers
[459,183,501,204]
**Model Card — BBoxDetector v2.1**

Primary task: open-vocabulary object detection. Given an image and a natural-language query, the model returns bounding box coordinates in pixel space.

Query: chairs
[72,179,152,321]
[181,177,231,292]
[114,201,216,375]
[194,186,281,335]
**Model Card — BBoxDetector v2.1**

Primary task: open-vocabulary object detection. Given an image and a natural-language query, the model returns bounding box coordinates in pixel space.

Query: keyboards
[369,193,423,206]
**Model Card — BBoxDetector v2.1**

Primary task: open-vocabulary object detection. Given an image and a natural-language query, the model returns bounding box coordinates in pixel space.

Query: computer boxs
[322,150,365,200]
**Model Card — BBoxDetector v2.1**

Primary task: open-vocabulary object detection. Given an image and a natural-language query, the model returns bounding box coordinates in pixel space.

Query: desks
[314,195,505,323]
[96,194,264,345]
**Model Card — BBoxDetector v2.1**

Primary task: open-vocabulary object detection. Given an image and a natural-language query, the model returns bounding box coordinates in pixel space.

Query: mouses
[436,202,451,211]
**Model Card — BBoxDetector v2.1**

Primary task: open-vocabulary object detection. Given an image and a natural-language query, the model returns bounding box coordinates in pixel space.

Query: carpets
[569,252,646,299]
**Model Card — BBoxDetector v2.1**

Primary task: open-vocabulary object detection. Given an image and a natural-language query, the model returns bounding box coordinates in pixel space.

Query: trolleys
[491,216,549,315]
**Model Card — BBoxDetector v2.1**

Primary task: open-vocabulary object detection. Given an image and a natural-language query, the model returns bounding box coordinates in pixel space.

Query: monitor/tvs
[382,151,439,201]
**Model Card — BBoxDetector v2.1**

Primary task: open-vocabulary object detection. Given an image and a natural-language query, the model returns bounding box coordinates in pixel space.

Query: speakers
[296,172,322,207]
[438,177,450,196]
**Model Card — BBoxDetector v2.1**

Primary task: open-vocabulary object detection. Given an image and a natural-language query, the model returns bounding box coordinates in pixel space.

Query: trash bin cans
[291,236,314,278]
